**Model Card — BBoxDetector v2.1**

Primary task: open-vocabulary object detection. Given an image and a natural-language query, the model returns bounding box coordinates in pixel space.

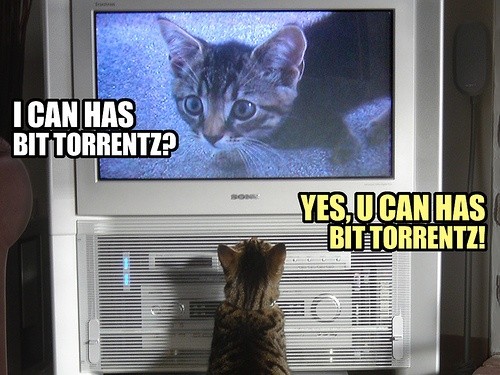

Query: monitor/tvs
[71,1,419,215]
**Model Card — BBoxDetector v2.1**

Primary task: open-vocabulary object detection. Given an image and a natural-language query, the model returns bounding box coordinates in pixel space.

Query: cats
[208,237,290,374]
[157,13,392,178]
